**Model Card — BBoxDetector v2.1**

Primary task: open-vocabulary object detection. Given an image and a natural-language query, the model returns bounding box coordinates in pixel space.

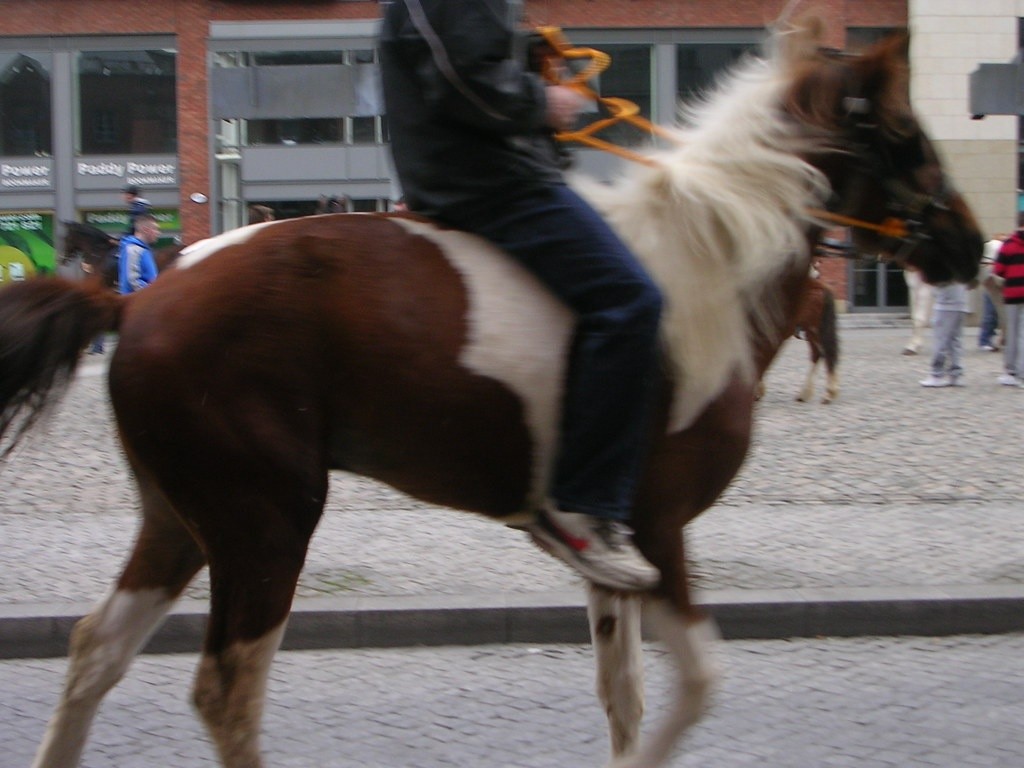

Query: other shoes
[978,343,998,351]
[946,376,965,386]
[905,345,917,354]
[919,376,944,387]
[1000,374,1019,386]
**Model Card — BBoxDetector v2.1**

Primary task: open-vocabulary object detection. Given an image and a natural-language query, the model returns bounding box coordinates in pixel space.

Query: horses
[750,279,842,408]
[57,216,188,295]
[0,16,989,767]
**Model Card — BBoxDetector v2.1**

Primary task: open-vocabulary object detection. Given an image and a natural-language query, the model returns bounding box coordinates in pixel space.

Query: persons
[246,191,408,226]
[121,186,152,235]
[904,211,1024,388]
[118,212,159,296]
[375,0,661,591]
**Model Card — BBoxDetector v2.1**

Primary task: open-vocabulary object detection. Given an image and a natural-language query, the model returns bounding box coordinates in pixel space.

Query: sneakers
[530,499,661,591]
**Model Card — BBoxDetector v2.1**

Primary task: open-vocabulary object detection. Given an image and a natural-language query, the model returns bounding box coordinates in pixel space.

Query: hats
[121,185,138,195]
